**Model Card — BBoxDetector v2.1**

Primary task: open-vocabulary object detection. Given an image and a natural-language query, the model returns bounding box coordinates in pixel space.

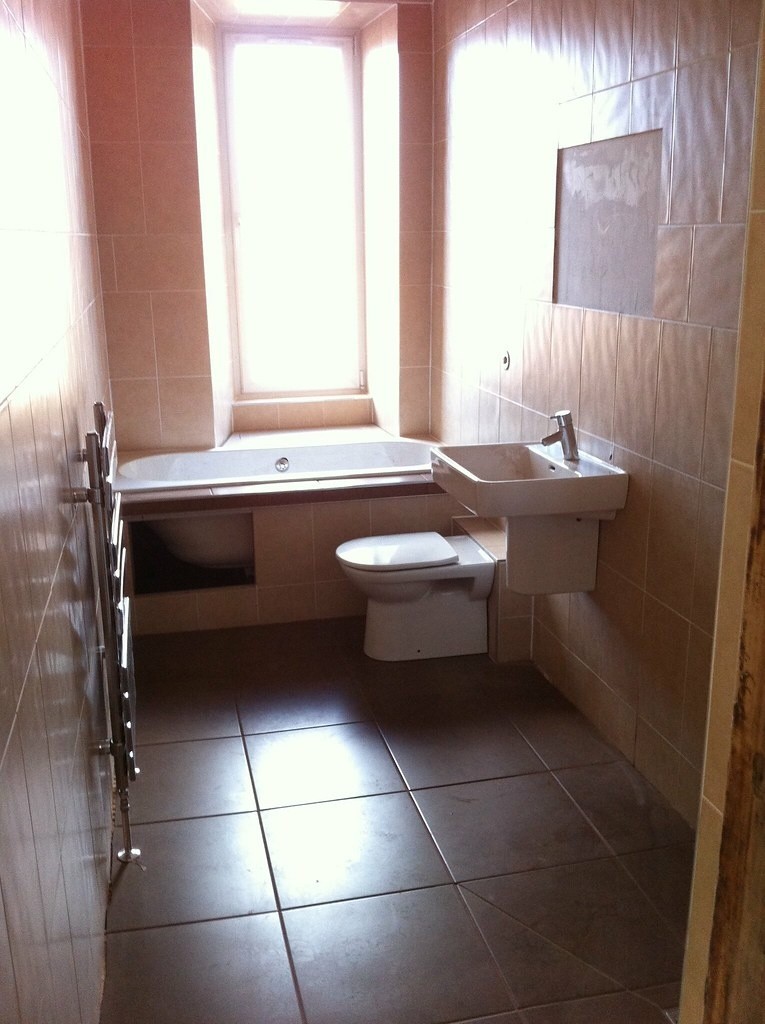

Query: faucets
[540,408,581,458]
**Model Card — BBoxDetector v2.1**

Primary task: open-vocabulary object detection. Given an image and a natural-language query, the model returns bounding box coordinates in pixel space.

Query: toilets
[334,532,497,662]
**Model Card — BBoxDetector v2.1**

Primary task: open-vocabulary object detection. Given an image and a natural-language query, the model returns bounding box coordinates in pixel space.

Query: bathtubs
[118,439,445,635]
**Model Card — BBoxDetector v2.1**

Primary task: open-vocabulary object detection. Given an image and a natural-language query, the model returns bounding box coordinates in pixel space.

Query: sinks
[432,440,628,516]
